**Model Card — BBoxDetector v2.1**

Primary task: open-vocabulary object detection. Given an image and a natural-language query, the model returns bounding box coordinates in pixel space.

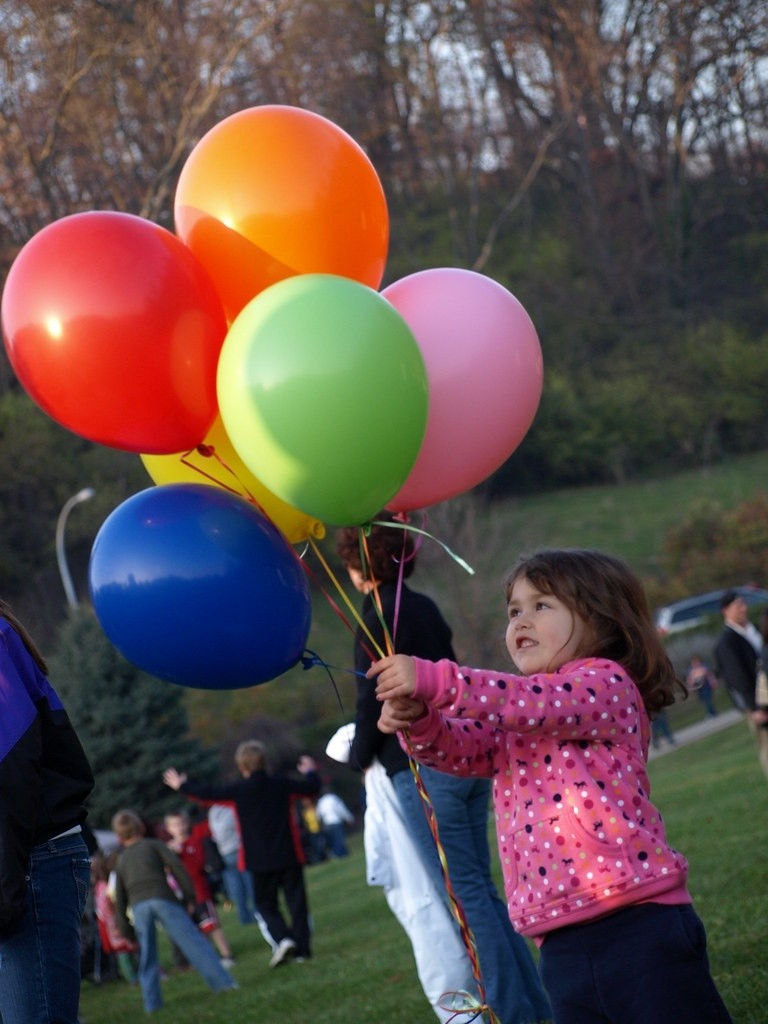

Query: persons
[337,518,551,1024]
[687,654,719,719]
[650,700,675,750]
[712,590,768,771]
[0,600,95,1024]
[366,551,732,1024]
[83,737,353,1016]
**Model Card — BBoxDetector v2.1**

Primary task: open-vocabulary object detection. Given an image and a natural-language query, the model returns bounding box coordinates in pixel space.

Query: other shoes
[219,954,240,971]
[268,937,306,969]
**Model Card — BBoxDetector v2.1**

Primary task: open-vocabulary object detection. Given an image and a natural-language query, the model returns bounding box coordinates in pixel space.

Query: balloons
[91,482,312,690]
[2,212,225,457]
[379,267,542,490]
[216,273,430,536]
[174,104,388,321]
[140,411,325,543]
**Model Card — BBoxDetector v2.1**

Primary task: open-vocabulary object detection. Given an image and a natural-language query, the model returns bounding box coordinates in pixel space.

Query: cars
[654,583,768,639]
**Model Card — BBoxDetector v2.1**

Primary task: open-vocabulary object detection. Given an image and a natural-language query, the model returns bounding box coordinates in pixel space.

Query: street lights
[53,487,96,612]
